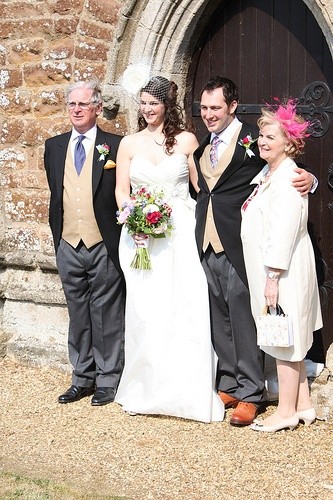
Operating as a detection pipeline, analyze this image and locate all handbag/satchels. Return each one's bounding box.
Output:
[256,302,293,347]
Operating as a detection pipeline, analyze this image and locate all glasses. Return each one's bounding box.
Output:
[67,100,89,109]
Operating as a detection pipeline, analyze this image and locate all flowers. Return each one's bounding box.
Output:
[116,184,172,270]
[96,143,110,162]
[102,158,116,170]
[238,133,260,161]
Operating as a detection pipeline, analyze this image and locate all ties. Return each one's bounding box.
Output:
[209,137,221,167]
[73,135,86,175]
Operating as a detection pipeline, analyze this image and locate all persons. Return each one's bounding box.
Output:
[114,76,226,423]
[239,97,323,431]
[194,77,332,426]
[44,82,132,405]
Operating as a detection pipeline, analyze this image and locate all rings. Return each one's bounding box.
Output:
[271,300,273,301]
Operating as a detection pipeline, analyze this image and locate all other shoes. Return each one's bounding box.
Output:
[263,378,280,401]
[303,359,323,377]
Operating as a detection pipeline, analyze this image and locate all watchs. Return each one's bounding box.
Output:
[267,272,280,279]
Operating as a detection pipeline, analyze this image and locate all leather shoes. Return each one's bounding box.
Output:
[90,387,114,405]
[217,391,237,408]
[59,386,93,403]
[229,401,257,425]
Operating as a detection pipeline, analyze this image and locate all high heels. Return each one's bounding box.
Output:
[296,407,316,427]
[250,413,298,431]
